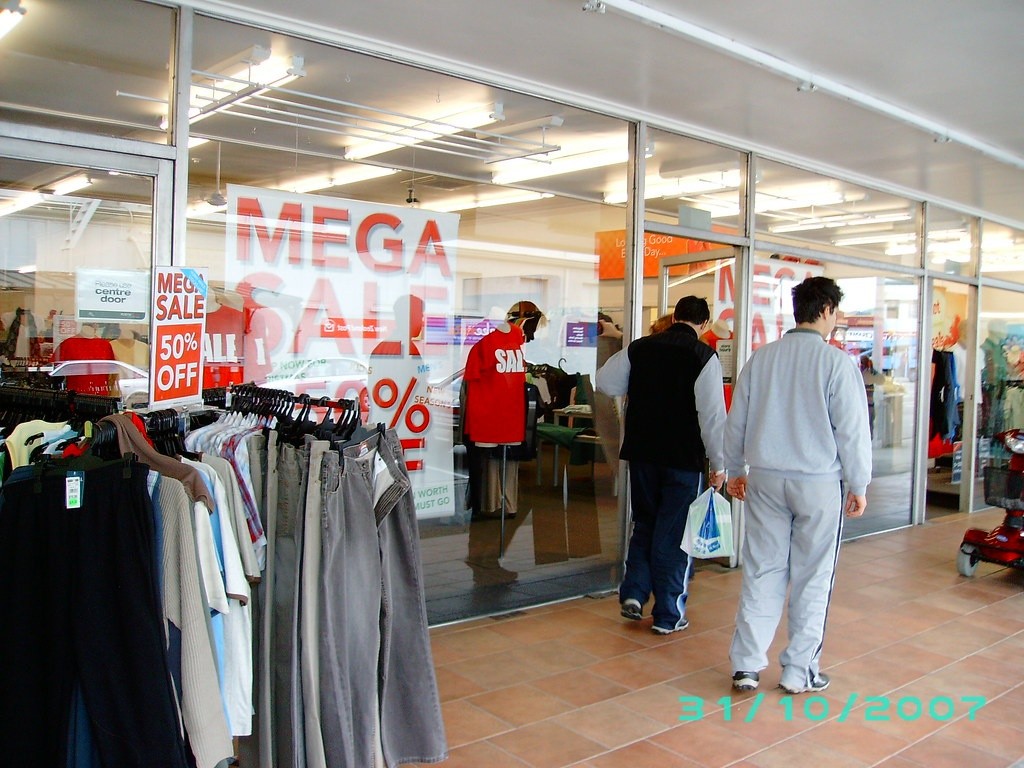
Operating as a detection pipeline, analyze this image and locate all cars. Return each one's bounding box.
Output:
[46,360,150,410]
[415,355,562,459]
[263,356,374,426]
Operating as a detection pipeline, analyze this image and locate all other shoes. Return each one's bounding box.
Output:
[473,567,518,584]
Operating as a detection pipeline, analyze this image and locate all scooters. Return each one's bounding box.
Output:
[954,428,1024,579]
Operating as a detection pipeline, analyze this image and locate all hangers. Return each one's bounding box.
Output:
[558,357,567,370]
[0,357,201,495]
[202,381,386,467]
[523,362,549,378]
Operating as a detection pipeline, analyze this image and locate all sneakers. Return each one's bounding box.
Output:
[620,598,644,620]
[802,672,830,693]
[731,671,760,690]
[650,618,690,634]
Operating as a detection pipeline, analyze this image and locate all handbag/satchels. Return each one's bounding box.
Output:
[679,486,735,559]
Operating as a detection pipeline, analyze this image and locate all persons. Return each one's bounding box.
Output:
[364,294,430,455]
[463,306,529,449]
[0,288,249,402]
[723,276,873,694]
[461,302,551,587]
[596,296,726,634]
